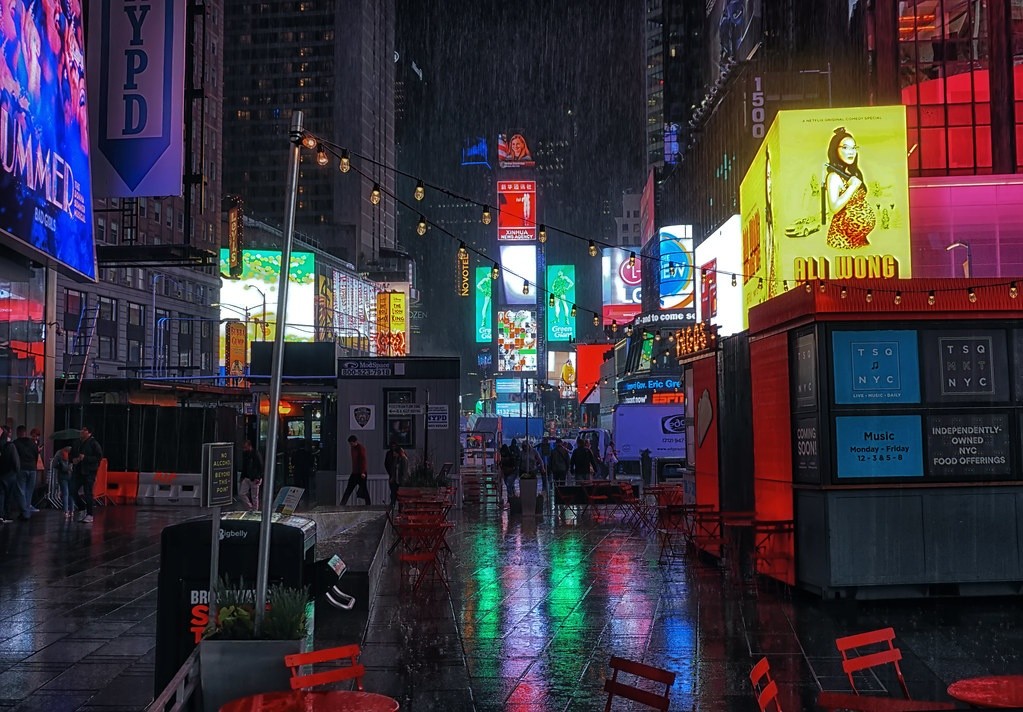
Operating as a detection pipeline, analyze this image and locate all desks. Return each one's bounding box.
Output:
[216,691,400,712]
[948,676,1023,709]
[720,518,759,582]
[656,502,716,566]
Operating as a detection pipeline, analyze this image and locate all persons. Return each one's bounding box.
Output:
[550,439,569,486]
[68,422,103,523]
[519,441,545,477]
[292,439,320,493]
[550,270,574,325]
[478,273,491,326]
[0,426,25,523]
[571,432,604,483]
[541,439,552,475]
[604,441,619,480]
[385,442,410,509]
[239,439,263,510]
[339,435,371,505]
[506,135,531,160]
[53,439,75,518]
[13,425,45,518]
[499,438,520,503]
[826,133,876,247]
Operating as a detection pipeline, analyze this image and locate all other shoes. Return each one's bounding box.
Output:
[82,515,93,523]
[77,510,87,521]
[0,513,14,523]
[29,505,40,512]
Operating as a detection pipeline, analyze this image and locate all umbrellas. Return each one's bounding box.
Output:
[50,429,83,439]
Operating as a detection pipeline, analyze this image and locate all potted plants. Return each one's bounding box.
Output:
[198,579,305,712]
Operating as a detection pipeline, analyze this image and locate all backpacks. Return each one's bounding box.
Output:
[0,442,15,476]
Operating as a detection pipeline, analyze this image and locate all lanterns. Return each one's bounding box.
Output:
[260,397,292,418]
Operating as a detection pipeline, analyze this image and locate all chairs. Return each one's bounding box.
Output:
[285,645,365,694]
[390,486,456,598]
[602,655,676,712]
[836,627,911,701]
[749,657,782,712]
[555,479,796,594]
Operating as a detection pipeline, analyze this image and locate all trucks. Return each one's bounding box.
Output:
[571,403,686,482]
[463,416,544,466]
